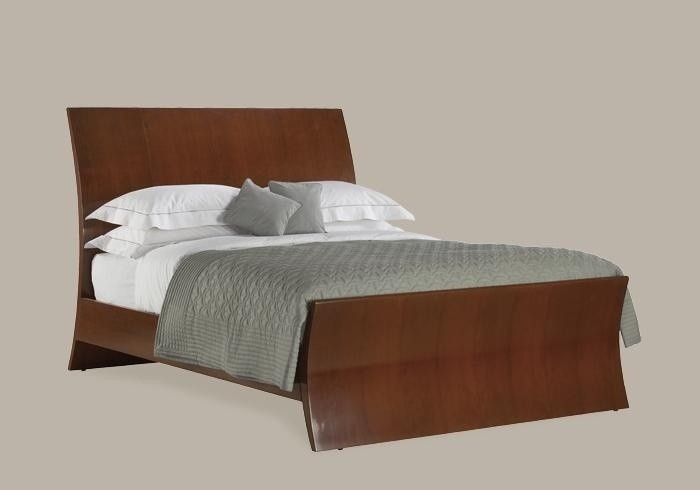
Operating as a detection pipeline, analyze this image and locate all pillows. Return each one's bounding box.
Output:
[82,225,240,262]
[265,178,416,220]
[222,177,305,239]
[84,182,242,231]
[269,179,329,237]
[320,219,405,236]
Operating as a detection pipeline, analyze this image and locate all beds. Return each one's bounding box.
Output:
[64,107,631,453]
